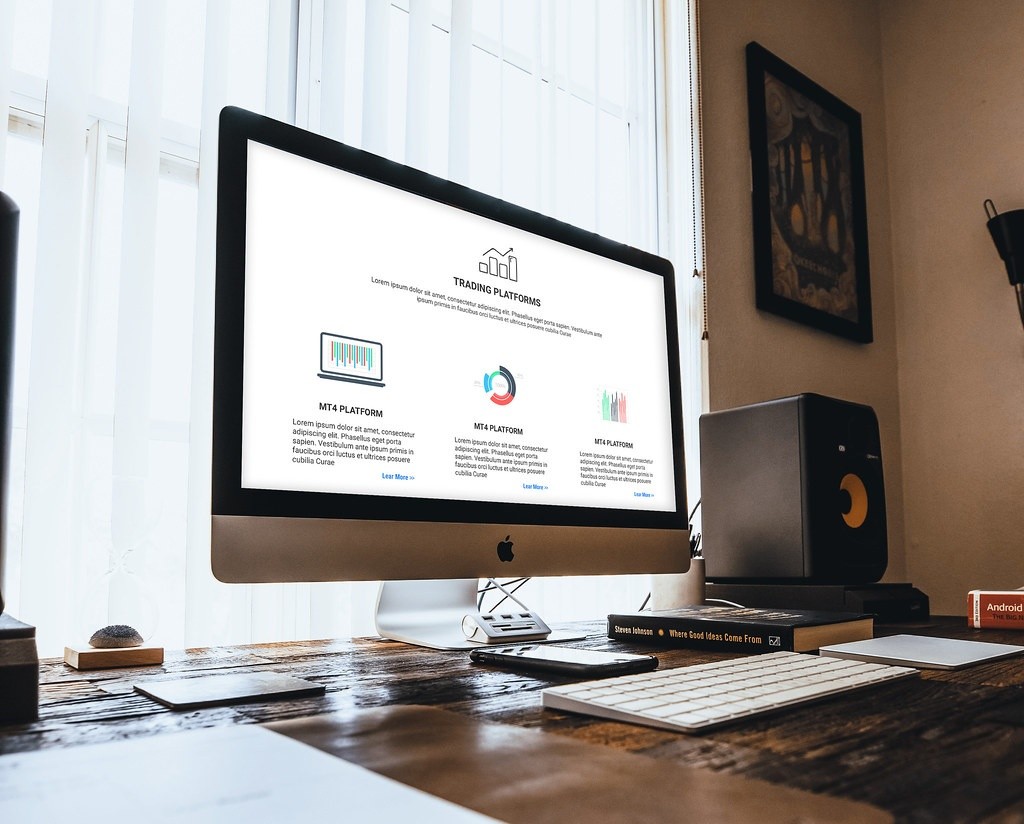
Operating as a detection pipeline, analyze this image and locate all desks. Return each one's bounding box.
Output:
[1,615,1024,824]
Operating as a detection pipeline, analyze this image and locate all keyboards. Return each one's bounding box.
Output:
[540,651,922,735]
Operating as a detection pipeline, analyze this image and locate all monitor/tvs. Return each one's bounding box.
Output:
[208,106,692,650]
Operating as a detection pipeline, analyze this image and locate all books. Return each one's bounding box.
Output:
[606,605,877,655]
[820,633,1024,671]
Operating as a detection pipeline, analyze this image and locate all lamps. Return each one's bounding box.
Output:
[982,198,1024,328]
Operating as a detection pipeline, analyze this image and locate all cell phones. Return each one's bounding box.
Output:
[470,644,659,680]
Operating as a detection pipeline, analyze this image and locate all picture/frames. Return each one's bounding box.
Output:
[745,40,874,346]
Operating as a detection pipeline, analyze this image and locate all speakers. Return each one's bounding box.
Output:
[699,392,890,585]
